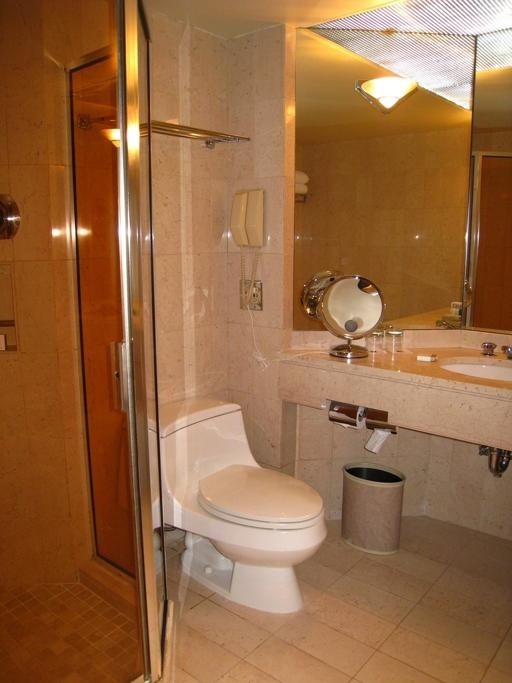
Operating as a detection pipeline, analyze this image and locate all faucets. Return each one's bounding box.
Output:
[501,346,512,359]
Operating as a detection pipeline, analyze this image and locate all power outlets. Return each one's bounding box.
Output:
[240,280,262,310]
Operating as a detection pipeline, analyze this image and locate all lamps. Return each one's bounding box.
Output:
[355,62,421,111]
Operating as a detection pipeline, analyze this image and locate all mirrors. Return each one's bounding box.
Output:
[292,27,512,335]
[319,275,384,358]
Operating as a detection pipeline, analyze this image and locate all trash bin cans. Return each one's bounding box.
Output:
[342,462,406,556]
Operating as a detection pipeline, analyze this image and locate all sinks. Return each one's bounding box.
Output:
[436,356,512,380]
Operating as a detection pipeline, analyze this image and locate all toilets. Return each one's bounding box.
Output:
[157,396,329,614]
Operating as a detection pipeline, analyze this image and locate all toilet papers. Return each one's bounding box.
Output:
[333,406,366,430]
